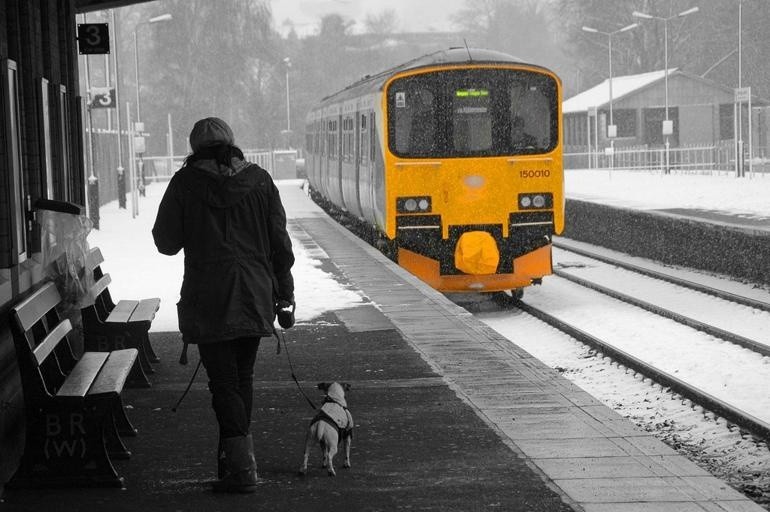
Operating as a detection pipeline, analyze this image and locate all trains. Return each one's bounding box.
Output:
[302,38,567,300]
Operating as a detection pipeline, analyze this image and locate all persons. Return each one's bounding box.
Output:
[151,115,298,494]
[513,115,538,150]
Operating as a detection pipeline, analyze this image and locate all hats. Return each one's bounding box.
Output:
[190,117,234,154]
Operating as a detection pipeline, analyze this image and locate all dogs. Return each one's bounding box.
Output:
[297,383,357,478]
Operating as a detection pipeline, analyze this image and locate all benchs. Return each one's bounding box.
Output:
[9,247,161,491]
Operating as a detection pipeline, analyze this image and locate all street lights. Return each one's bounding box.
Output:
[582,21,640,170]
[126,13,175,234]
[630,5,705,176]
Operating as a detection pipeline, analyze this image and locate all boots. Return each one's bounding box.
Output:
[212,431,260,493]
